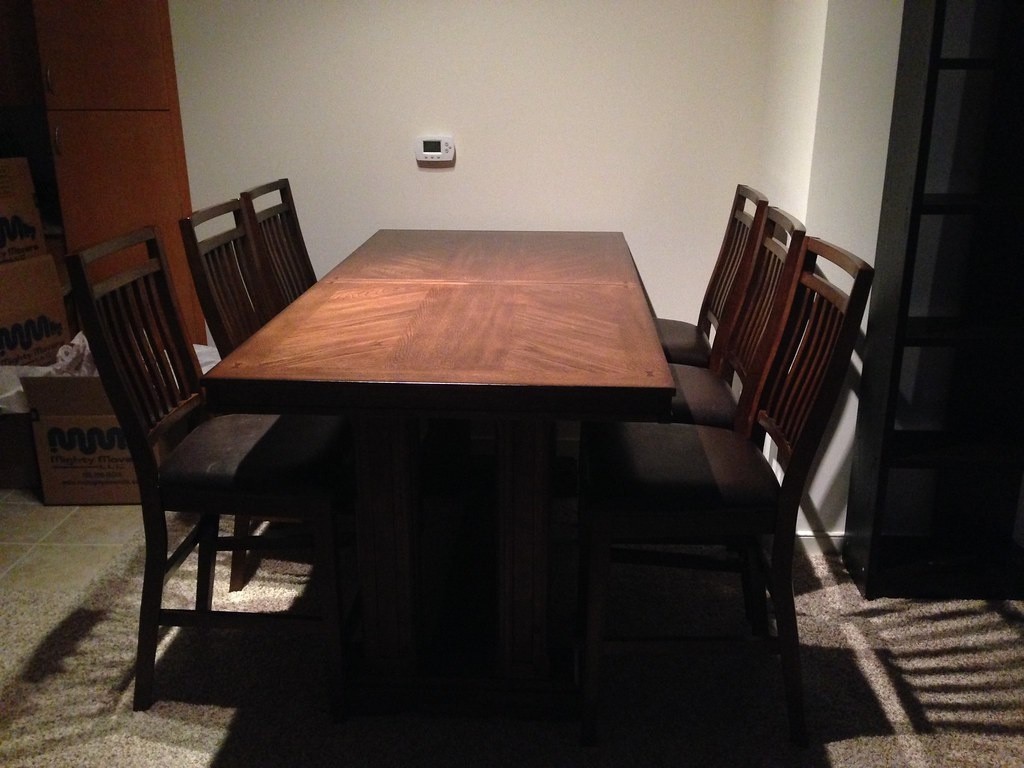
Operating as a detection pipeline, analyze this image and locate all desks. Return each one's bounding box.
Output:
[199,228,676,716]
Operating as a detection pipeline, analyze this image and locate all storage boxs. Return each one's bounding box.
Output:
[0,159,139,507]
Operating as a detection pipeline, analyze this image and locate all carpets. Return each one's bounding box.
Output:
[0,515,1024,766]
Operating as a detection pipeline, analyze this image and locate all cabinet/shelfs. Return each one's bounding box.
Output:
[33,0,206,344]
[861,0,1024,600]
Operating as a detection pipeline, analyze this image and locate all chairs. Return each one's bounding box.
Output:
[177,198,280,364]
[567,235,879,696]
[69,224,363,721]
[584,207,810,426]
[654,183,770,369]
[239,179,319,303]
